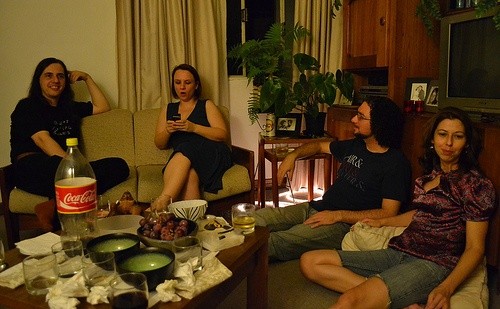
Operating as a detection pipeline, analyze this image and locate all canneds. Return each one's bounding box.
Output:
[265,113,275,137]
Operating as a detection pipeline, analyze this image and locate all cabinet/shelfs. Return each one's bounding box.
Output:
[326,0,500,269]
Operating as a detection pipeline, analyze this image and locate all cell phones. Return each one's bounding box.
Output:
[172,114,181,121]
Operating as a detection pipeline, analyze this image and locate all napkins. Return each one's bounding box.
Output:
[0,232,113,309]
[196,229,245,251]
[147,255,198,307]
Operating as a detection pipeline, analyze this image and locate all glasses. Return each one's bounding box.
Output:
[356,112,370,120]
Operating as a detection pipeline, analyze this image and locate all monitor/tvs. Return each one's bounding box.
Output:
[440,4,500,114]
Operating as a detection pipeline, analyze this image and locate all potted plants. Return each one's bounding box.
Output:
[227,20,354,138]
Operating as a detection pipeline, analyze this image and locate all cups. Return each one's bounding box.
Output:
[415,101,424,113]
[51,241,84,284]
[23,252,58,296]
[151,194,172,223]
[231,203,256,235]
[403,100,414,112]
[109,272,149,309]
[81,251,117,291]
[172,237,202,273]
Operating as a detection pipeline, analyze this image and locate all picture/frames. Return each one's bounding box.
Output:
[276,113,303,138]
[404,77,429,103]
[423,80,440,112]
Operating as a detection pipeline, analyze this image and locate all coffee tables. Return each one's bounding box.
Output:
[0,227,272,309]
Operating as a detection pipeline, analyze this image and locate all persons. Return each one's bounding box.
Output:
[254,97,413,267]
[414,86,438,105]
[300,108,494,309]
[147,64,225,217]
[279,120,294,131]
[10,58,130,236]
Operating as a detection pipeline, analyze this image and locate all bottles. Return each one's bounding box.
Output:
[265,112,276,137]
[55,138,99,240]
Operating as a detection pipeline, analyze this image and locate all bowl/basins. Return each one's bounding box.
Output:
[99,214,145,234]
[168,200,208,222]
[137,218,199,249]
[87,233,141,270]
[116,247,175,293]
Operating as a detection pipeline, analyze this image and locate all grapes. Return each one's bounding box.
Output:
[139,213,188,241]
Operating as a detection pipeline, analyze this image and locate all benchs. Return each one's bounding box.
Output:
[0,105,255,249]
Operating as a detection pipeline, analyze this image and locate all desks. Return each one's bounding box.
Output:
[255,132,336,209]
